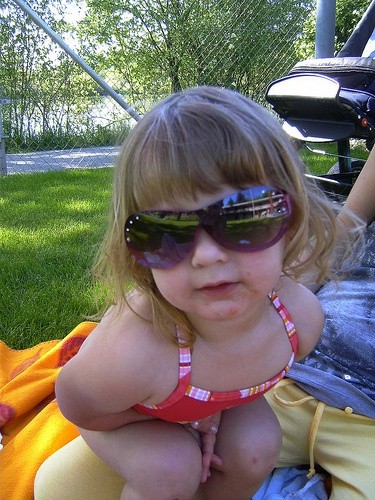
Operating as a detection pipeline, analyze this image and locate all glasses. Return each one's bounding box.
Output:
[123,187,297,271]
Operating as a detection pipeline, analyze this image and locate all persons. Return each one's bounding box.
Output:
[55,85,362,500]
[34,144,375,500]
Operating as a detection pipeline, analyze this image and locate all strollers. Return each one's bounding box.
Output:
[262,0,375,203]
